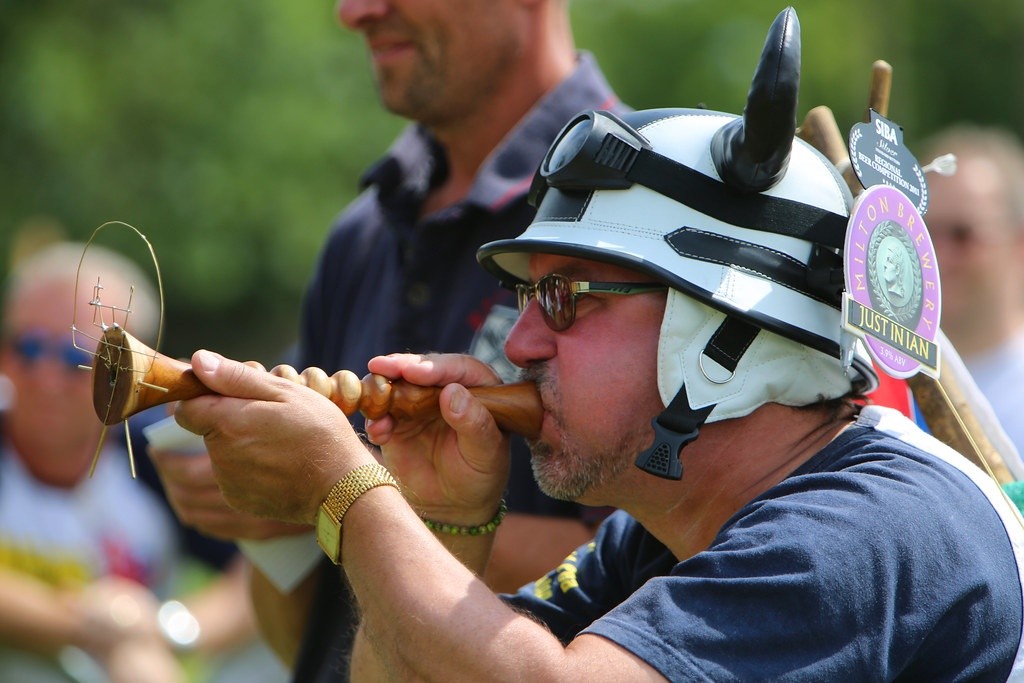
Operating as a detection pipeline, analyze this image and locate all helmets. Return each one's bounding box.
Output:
[477,6,880,479]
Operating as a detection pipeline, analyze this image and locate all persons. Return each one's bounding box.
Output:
[911,124,1024,470]
[146,0,637,683]
[0,241,290,683]
[174,4,1024,683]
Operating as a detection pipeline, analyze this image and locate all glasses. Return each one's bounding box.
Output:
[515,274,669,332]
[16,342,101,370]
[527,110,847,251]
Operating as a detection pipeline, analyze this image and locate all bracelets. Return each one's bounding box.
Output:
[422,498,507,535]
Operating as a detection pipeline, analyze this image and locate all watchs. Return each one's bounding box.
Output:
[317,464,401,565]
